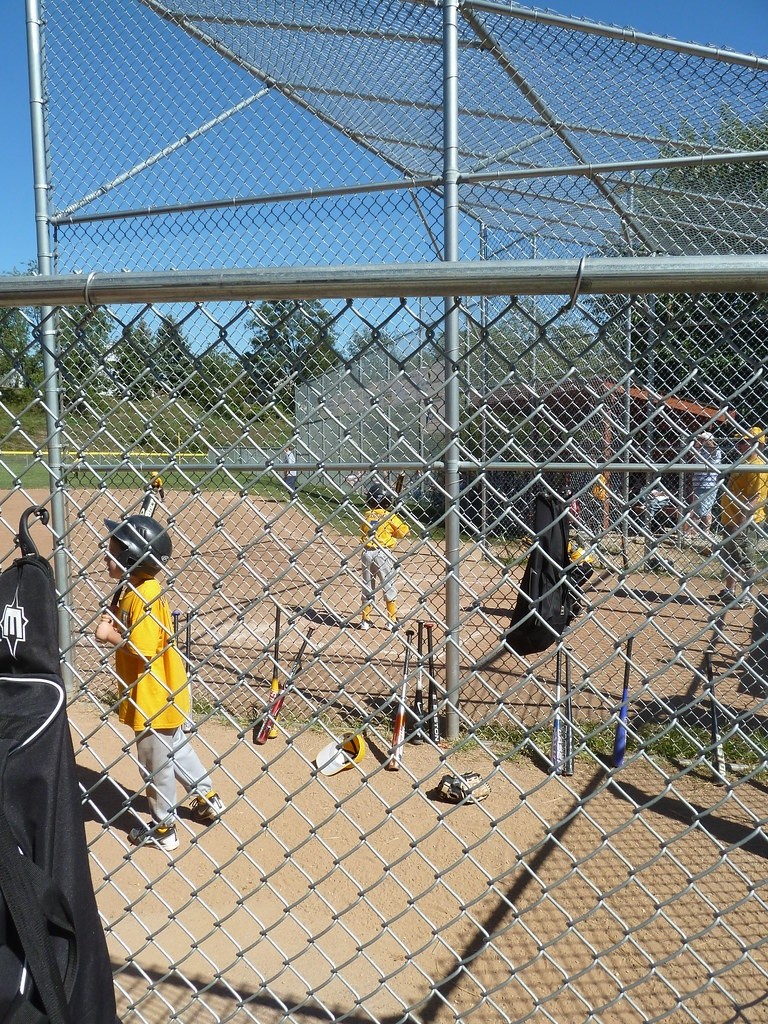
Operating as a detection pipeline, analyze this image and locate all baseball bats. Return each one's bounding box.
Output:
[95,488,160,645]
[423,623,440,745]
[171,610,193,733]
[551,639,574,777]
[703,650,727,787]
[388,629,414,771]
[411,619,425,745]
[254,603,315,745]
[393,471,406,515]
[611,633,635,767]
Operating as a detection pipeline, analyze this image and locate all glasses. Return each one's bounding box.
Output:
[738,437,747,443]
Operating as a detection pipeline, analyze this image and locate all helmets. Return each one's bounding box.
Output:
[366,483,392,509]
[104,515,172,577]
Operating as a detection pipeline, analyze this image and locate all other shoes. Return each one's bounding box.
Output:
[576,608,583,619]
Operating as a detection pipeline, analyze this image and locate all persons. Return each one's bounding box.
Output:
[149,472,165,500]
[683,435,721,539]
[359,483,411,631]
[284,447,298,502]
[96,515,225,850]
[706,427,768,607]
[567,542,594,617]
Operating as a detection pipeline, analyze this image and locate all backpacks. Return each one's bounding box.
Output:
[505,488,594,656]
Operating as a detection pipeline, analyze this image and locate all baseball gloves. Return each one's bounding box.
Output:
[520,537,533,551]
[435,770,491,804]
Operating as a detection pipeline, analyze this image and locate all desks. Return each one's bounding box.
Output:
[631,506,678,530]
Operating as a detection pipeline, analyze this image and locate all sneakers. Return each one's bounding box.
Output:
[709,589,735,600]
[188,792,225,822]
[725,594,753,609]
[129,820,180,852]
[385,621,405,630]
[360,618,374,630]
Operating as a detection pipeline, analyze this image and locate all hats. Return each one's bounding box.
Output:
[734,426,765,444]
[314,733,365,776]
[698,432,714,441]
